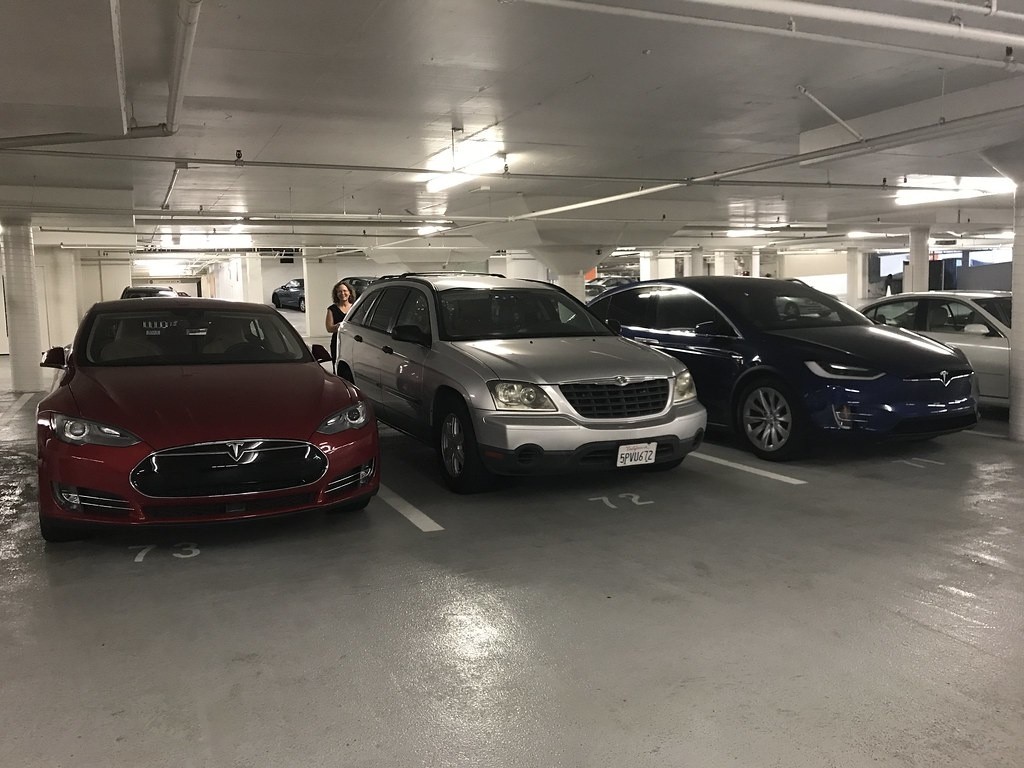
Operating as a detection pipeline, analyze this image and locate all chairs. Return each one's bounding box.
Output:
[100,317,254,360]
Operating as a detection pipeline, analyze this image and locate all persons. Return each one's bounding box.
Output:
[327,283,359,373]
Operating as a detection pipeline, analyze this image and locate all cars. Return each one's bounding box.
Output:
[34,296,380,541]
[339,274,380,298]
[585,277,634,295]
[585,275,982,461]
[334,271,708,495]
[271,278,306,312]
[856,290,1012,408]
[120,285,180,300]
[775,278,838,316]
